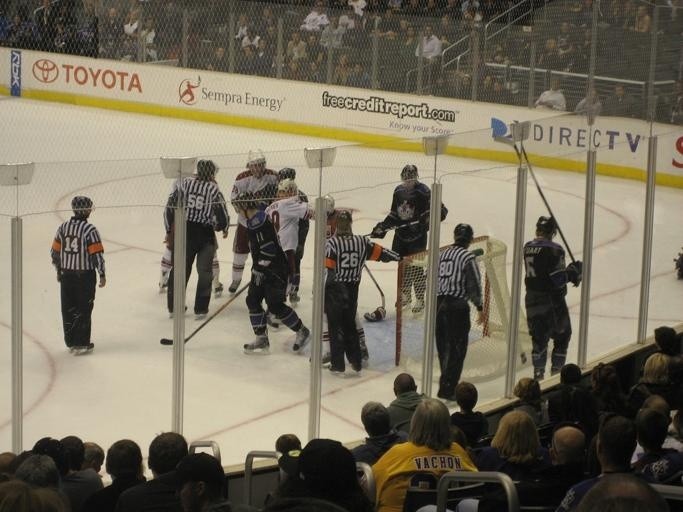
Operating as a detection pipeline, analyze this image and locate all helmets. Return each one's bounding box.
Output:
[536,217,558,233]
[72,197,93,218]
[277,167,298,196]
[246,149,266,164]
[337,209,353,230]
[197,159,215,178]
[454,224,473,245]
[325,195,335,212]
[401,164,418,180]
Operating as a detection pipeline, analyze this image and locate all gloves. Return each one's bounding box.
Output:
[566,260,582,287]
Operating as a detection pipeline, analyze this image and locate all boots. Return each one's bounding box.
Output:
[293,327,310,350]
[229,281,240,291]
[159,276,170,290]
[326,363,346,374]
[243,334,271,350]
[289,288,300,301]
[215,283,223,297]
[438,389,462,400]
[351,363,365,373]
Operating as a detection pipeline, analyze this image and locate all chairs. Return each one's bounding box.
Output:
[0,1,682,128]
[253,350,682,512]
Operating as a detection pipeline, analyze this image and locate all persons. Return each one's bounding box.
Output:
[524,216,582,382]
[1,0,682,126]
[50,196,106,354]
[1,327,683,511]
[162,156,485,404]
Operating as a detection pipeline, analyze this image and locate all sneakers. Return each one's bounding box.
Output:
[66,343,94,350]
[395,293,425,313]
[167,303,189,316]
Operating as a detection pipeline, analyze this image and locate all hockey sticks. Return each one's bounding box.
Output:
[160,280,252,345]
[495,135,575,264]
[364,264,385,308]
